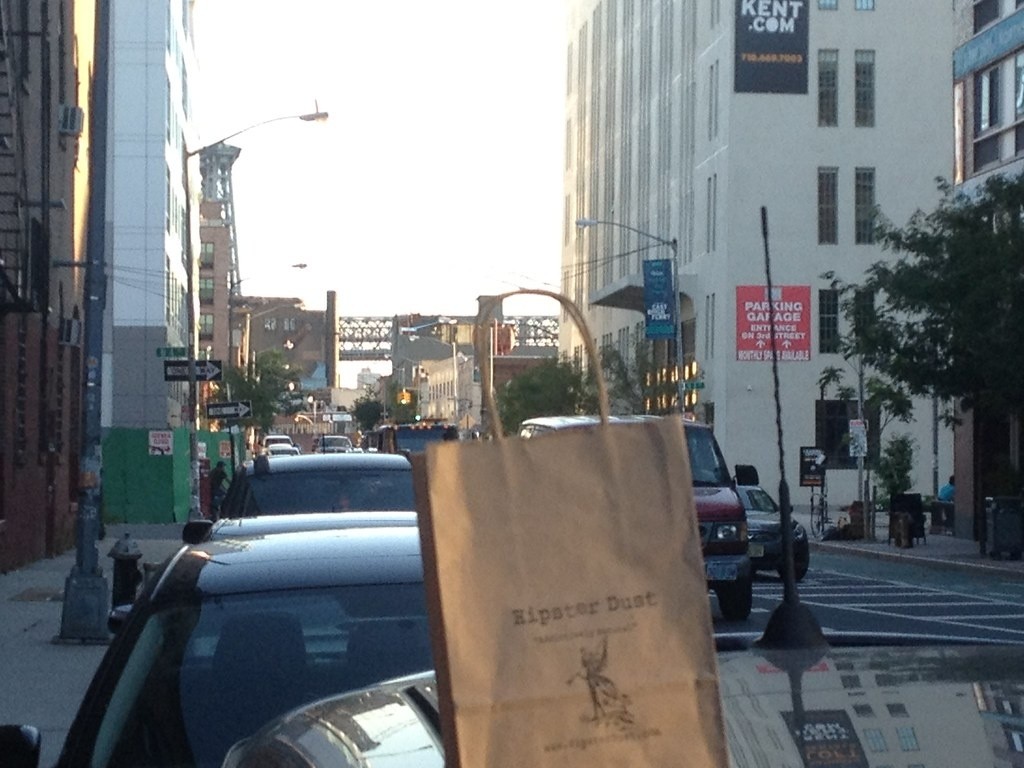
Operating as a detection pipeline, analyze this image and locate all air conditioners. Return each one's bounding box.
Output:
[59,104,83,136]
[59,317,80,345]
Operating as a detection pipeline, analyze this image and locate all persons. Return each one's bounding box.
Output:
[937,474,955,505]
[207,461,232,523]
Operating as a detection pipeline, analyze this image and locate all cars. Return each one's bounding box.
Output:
[0,511,444,768]
[222,647,1024,768]
[216,452,418,521]
[315,435,353,453]
[258,435,302,458]
[735,484,811,584]
[680,420,760,624]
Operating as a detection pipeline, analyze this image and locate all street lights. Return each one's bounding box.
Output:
[228,264,307,482]
[575,219,687,416]
[182,100,330,521]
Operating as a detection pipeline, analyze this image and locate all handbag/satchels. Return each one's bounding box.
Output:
[412,288,731,768]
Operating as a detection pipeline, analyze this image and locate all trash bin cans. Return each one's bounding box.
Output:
[984,494,1024,559]
